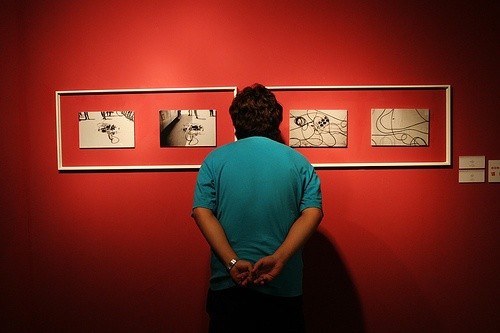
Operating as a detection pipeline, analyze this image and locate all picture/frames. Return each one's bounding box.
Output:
[55,87,237,170]
[264,85,451,167]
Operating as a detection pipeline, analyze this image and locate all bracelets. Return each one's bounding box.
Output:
[226,257,240,273]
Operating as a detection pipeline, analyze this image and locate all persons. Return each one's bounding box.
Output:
[191,83,324,333]
